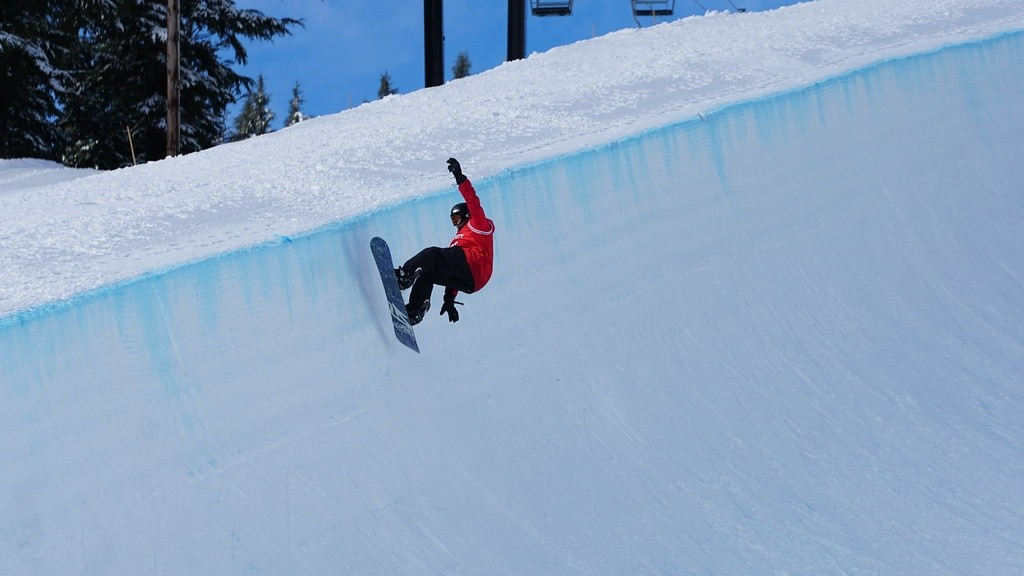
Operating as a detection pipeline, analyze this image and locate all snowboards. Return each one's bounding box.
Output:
[366,236,421,351]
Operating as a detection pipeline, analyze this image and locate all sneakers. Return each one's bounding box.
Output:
[394,266,414,290]
[409,299,431,326]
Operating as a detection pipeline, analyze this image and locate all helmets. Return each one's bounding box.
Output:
[449,203,470,226]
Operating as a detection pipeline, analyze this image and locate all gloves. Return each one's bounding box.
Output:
[446,158,467,185]
[440,294,464,323]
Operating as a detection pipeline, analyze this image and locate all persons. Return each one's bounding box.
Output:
[393,156,496,328]
